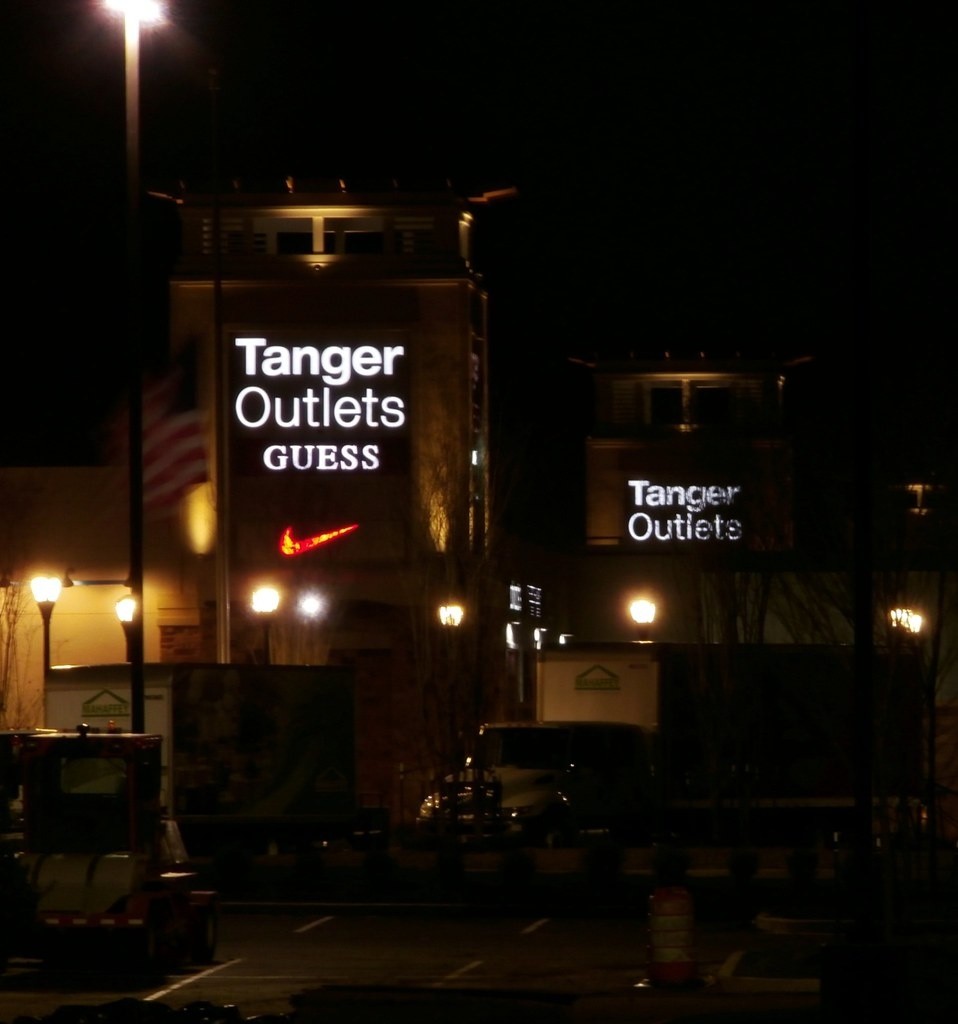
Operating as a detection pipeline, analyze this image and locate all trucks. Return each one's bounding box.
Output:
[416,640,926,848]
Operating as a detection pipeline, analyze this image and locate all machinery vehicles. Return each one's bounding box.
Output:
[0,737,220,990]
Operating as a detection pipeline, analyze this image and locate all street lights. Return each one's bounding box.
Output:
[104,2,154,734]
[32,576,62,670]
[116,599,132,663]
[252,588,278,663]
